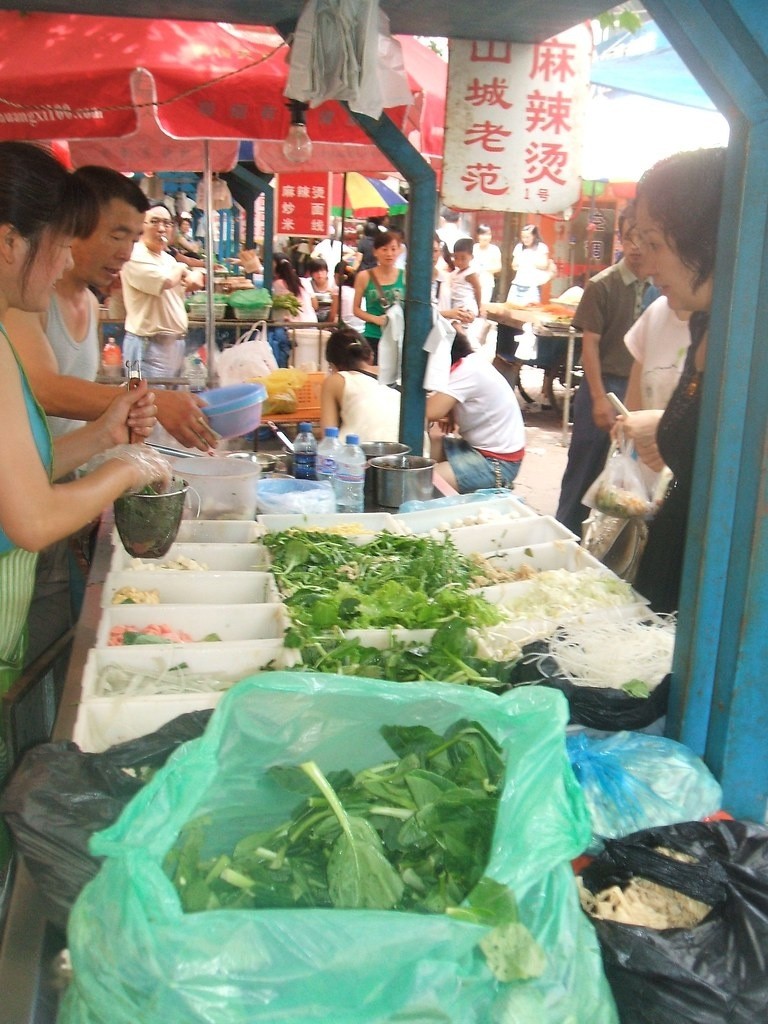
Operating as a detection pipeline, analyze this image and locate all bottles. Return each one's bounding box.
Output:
[316,427,344,489]
[181,358,207,390]
[333,434,366,513]
[103,337,121,378]
[293,423,318,480]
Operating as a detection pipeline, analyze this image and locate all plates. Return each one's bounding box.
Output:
[226,452,278,470]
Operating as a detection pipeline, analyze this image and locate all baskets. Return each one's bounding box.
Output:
[188,302,228,320]
[232,300,272,319]
[293,371,327,411]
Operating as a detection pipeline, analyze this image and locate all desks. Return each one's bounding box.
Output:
[487,304,583,447]
[0,450,720,1024]
[98,319,339,375]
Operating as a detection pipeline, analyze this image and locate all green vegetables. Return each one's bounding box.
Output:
[168,716,548,987]
[271,291,304,317]
[119,527,508,688]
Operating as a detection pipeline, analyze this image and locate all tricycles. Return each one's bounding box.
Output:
[486,298,588,425]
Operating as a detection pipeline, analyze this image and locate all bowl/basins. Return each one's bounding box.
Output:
[196,382,268,441]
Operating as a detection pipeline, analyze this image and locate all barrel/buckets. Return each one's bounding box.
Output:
[361,441,413,486]
[171,457,261,523]
[368,455,437,508]
[288,329,332,373]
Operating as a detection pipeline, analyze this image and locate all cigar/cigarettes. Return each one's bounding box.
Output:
[161,237,168,241]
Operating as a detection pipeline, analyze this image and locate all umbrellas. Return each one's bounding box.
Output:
[0,8,451,174]
[331,172,410,219]
[578,168,638,288]
[591,21,716,109]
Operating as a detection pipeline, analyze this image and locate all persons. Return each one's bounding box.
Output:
[0,139,206,989]
[225,148,729,621]
[321,328,401,444]
[450,238,482,337]
[472,225,501,303]
[506,225,549,304]
[426,333,525,491]
[556,207,666,537]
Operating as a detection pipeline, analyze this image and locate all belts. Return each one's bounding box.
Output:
[144,332,186,342]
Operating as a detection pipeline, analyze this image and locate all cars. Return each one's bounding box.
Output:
[289,239,325,278]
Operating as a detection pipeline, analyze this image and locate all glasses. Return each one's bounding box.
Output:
[145,219,175,226]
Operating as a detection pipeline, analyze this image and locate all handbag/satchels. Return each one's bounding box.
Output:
[581,436,666,516]
[1,709,216,930]
[568,729,723,854]
[246,368,306,416]
[215,320,277,387]
[61,668,616,1023]
[577,819,768,1024]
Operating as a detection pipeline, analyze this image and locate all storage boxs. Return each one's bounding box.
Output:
[110,544,272,575]
[68,695,222,797]
[79,640,303,710]
[95,602,293,650]
[258,496,676,687]
[102,569,278,608]
[111,519,261,548]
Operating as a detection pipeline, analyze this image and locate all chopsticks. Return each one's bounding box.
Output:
[606,392,630,417]
